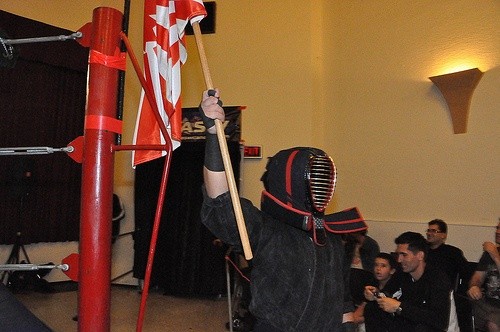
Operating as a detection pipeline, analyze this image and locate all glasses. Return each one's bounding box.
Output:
[426,227,444,234]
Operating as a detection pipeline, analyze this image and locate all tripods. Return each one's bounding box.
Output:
[0,197,31,287]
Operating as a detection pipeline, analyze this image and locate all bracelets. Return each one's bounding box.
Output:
[393,306,402,317]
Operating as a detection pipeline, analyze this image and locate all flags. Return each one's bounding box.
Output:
[132,0,207,169]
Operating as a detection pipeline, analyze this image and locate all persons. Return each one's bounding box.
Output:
[199,88,368,332]
[342,220,500,332]
[72,192,126,321]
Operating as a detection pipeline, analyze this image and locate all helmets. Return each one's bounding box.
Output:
[266,147,337,218]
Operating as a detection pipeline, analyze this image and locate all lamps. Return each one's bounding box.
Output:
[429,67,484,135]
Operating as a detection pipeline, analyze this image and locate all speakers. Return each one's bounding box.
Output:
[7,158,34,197]
